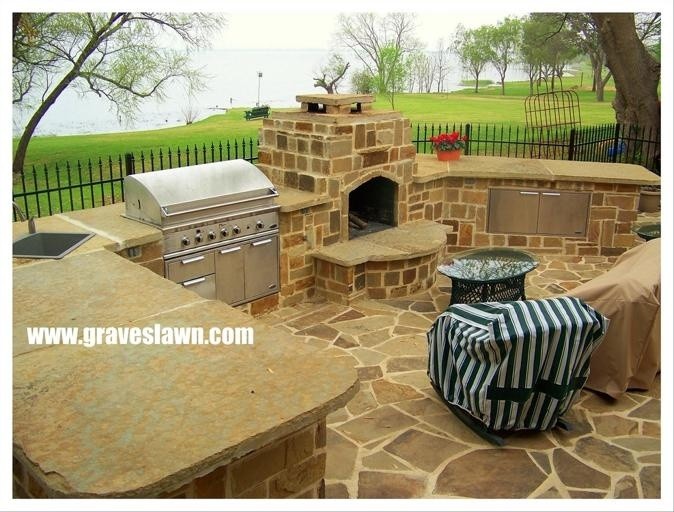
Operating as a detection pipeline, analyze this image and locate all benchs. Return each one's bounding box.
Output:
[245,106,270,121]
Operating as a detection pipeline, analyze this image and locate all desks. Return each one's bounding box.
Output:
[437,245,542,306]
[630,221,661,242]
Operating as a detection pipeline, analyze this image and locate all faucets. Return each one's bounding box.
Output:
[13,202,25,222]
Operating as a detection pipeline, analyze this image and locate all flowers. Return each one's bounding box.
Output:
[429,132,467,154]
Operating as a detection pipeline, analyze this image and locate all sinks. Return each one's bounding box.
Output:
[13,232,96,259]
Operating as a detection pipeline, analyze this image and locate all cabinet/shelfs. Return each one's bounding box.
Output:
[488,188,590,237]
[215,232,280,307]
[165,249,215,300]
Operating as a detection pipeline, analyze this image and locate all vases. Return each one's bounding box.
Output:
[436,148,461,161]
